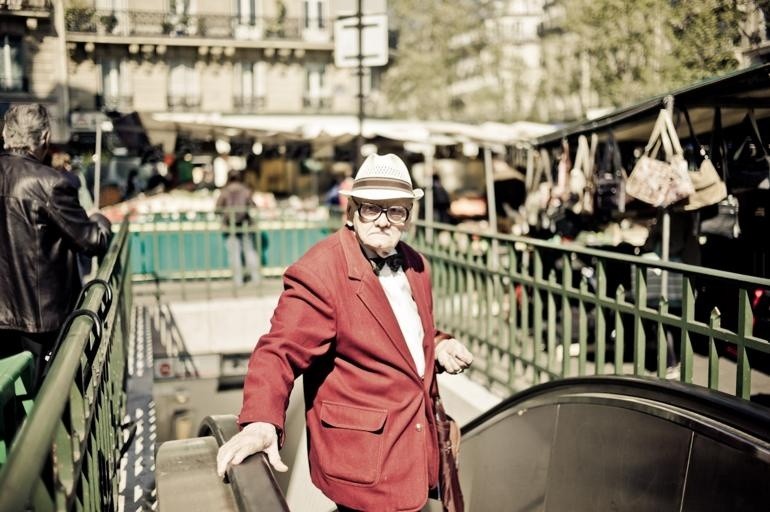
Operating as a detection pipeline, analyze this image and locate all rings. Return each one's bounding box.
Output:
[456,369,463,373]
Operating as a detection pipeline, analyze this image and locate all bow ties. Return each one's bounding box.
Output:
[369,254,403,273]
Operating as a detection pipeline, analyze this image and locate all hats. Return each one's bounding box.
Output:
[334,153,427,201]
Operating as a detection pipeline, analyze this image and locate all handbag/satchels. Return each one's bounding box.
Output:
[515,131,619,230]
[698,194,744,240]
[629,256,688,304]
[657,154,696,208]
[680,154,729,212]
[433,397,465,512]
[625,154,676,207]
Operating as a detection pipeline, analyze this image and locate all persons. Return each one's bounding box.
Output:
[46,139,454,229]
[214,149,479,512]
[214,167,263,291]
[0,96,113,439]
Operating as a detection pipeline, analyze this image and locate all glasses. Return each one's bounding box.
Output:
[350,195,415,225]
